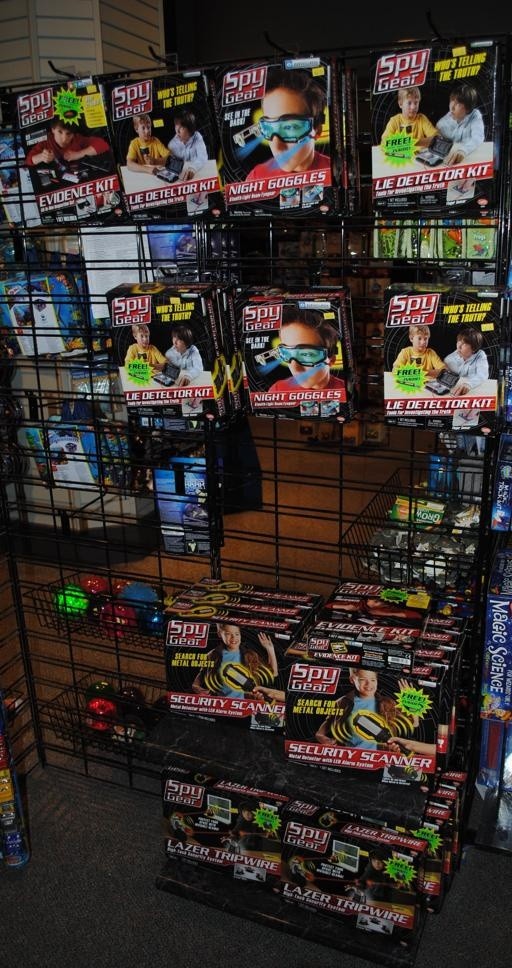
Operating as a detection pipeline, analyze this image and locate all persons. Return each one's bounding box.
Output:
[442,326,489,397]
[220,800,273,852]
[381,84,442,154]
[384,736,436,756]
[267,308,344,392]
[192,622,280,700]
[125,113,174,175]
[243,71,333,194]
[392,324,449,386]
[315,668,419,752]
[25,118,110,169]
[165,326,204,387]
[167,109,210,182]
[125,321,171,379]
[351,849,400,903]
[432,84,487,166]
[252,685,286,702]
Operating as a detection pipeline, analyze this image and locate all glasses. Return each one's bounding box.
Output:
[277,344,338,368]
[259,113,317,144]
